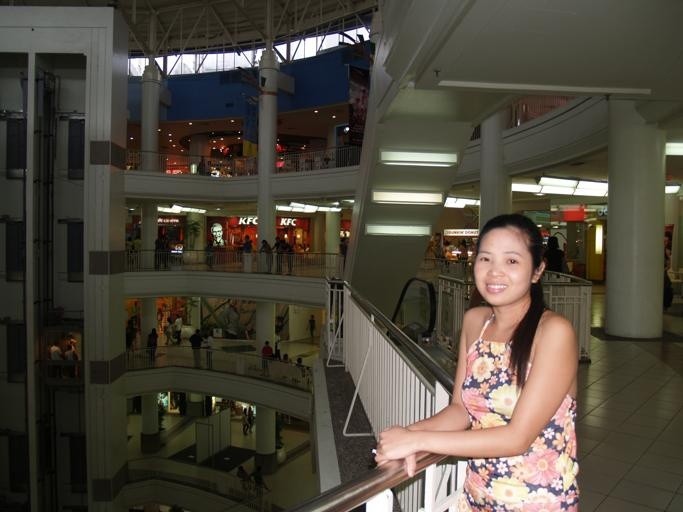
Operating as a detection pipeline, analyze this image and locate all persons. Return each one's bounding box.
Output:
[238,466,267,500]
[126,228,311,277]
[194,153,207,175]
[664,231,672,250]
[241,407,255,435]
[372,212,581,511]
[124,312,311,388]
[425,229,476,275]
[661,245,673,312]
[48,334,78,361]
[178,397,187,416]
[542,236,566,277]
[158,406,165,431]
